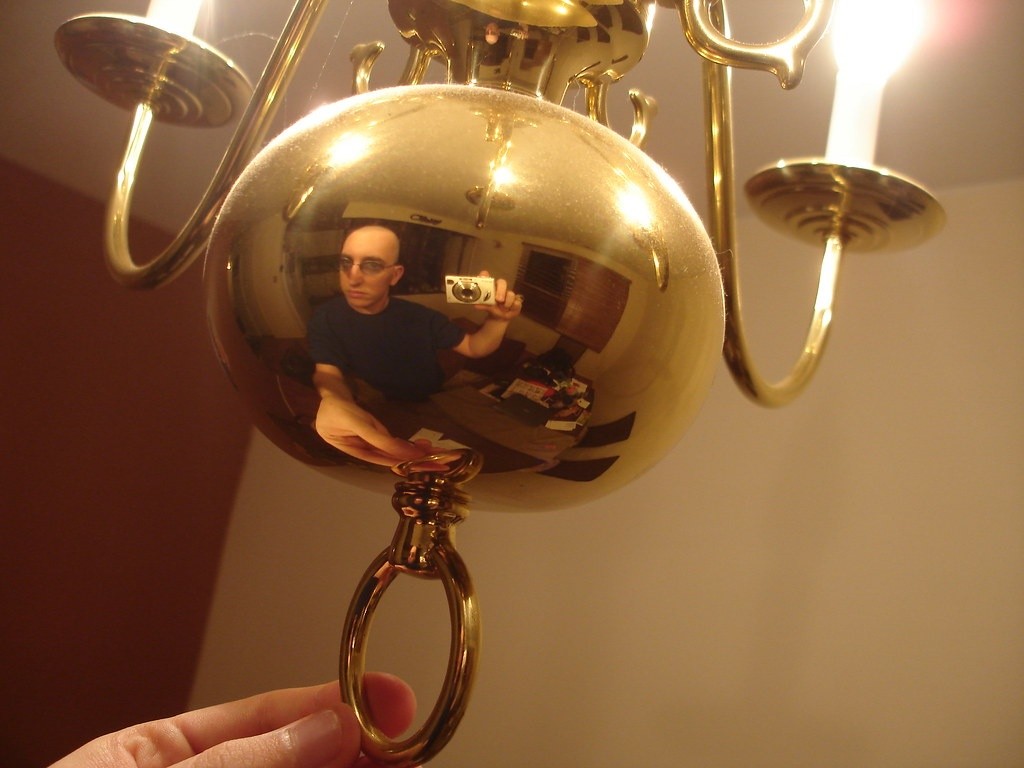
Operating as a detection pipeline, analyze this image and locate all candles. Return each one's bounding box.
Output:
[147,0,203,35]
[827,56,882,168]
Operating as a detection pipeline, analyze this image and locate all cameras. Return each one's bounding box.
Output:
[445,276,496,305]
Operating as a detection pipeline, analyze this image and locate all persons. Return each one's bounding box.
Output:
[45,672,424,768]
[310,225,523,471]
